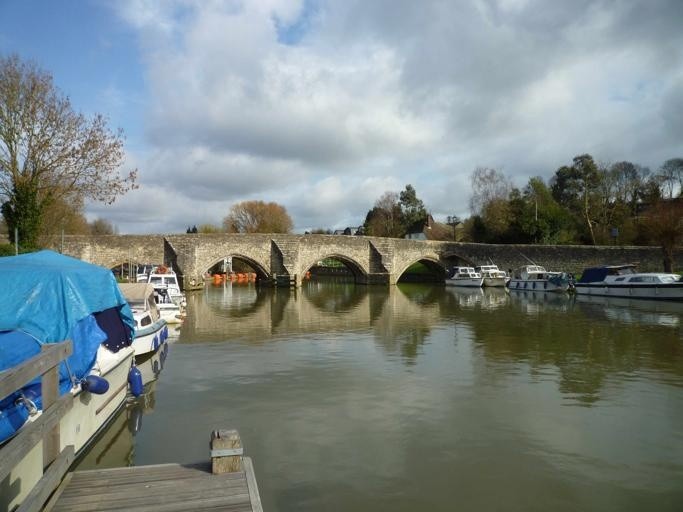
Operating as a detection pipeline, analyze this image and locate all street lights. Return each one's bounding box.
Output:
[446,216,458,240]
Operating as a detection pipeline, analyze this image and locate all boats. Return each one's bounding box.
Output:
[445,254,572,292]
[110,265,185,385]
[572,264,681,300]
[1,251,135,510]
